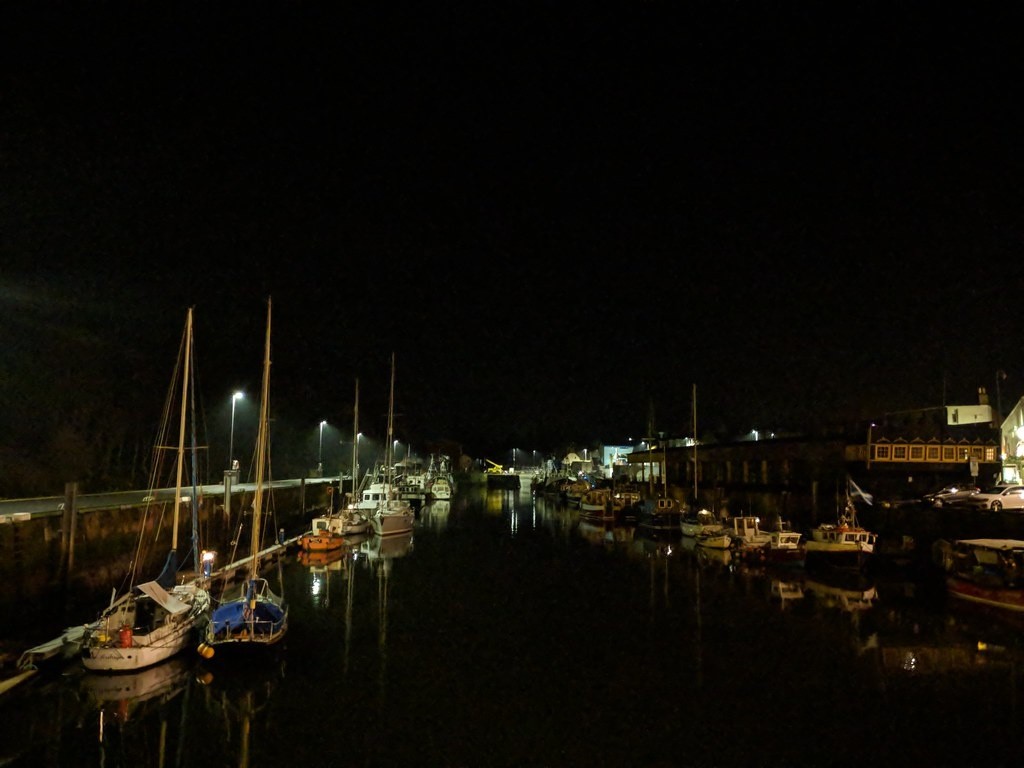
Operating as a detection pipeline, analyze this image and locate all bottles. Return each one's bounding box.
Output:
[119,623,133,648]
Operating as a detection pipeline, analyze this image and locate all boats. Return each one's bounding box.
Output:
[537,475,802,561]
[924,536,1024,626]
[302,374,457,551]
[801,521,877,565]
[76,307,225,673]
[203,295,295,644]
